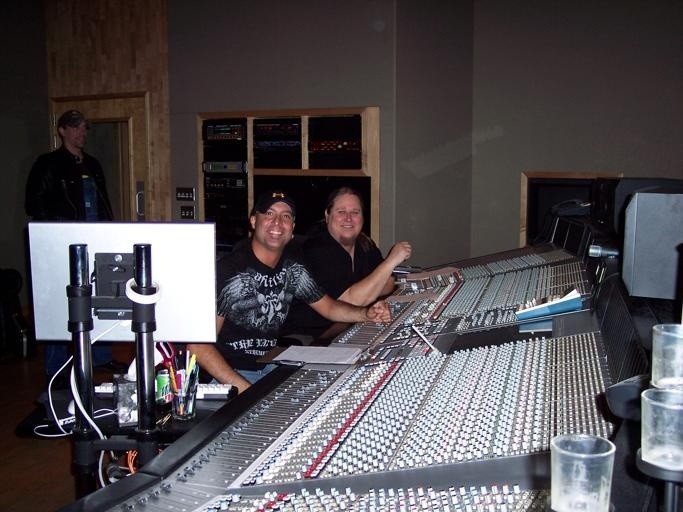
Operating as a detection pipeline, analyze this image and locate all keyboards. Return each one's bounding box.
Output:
[95,381,233,401]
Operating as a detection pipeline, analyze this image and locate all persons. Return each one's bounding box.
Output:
[183,184,394,401]
[21,108,127,391]
[285,184,412,349]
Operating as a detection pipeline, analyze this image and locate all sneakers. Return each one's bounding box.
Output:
[94,359,128,374]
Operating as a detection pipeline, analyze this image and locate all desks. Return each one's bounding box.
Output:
[16,360,228,499]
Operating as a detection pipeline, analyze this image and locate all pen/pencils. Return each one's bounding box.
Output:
[412,324,436,350]
[168,350,199,416]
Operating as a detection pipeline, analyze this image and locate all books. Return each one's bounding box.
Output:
[515,287,583,321]
[518,320,552,333]
[271,345,362,364]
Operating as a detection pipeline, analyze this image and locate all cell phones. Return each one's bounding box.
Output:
[279,360,305,367]
[395,265,422,272]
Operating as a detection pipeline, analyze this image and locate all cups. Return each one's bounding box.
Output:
[551,325,683,512]
[171,385,198,420]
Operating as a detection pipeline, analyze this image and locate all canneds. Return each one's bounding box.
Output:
[156,369,174,405]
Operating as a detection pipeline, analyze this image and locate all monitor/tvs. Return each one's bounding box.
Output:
[28,222,217,345]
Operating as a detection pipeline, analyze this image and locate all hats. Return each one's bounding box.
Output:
[248,189,295,217]
[56,110,88,129]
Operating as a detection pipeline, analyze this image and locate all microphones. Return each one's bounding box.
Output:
[588,244,620,258]
